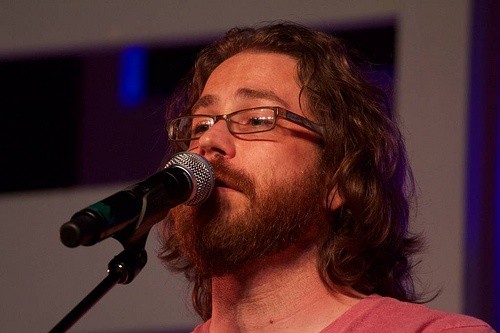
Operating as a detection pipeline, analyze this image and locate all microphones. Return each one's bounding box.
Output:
[59,152,215,249]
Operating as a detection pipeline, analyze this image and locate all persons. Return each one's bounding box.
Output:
[154,23,496,333]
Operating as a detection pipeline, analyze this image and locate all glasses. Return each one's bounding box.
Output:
[164,104,335,146]
